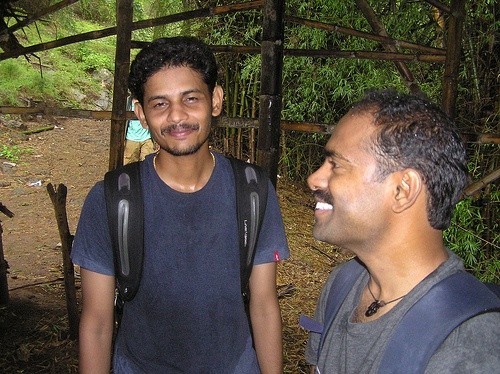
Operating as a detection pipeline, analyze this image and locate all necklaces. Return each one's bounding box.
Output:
[152,151,215,171]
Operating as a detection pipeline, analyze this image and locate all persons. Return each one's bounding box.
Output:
[300,94,499,374]
[70,34,294,374]
[365,279,408,317]
